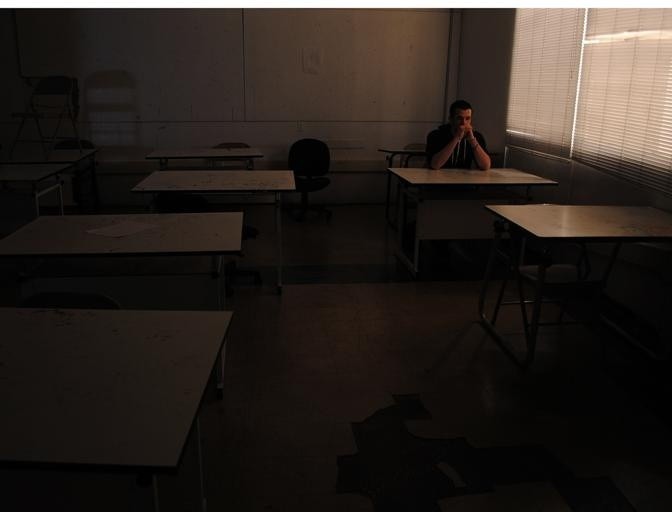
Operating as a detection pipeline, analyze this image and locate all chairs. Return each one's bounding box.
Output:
[287,138,333,223]
[10,75,85,157]
[209,142,255,170]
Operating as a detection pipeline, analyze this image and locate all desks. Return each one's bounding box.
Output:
[2,310,233,512]
[379,145,427,225]
[387,165,562,285]
[476,204,672,366]
[2,146,99,217]
[132,169,296,299]
[2,212,245,314]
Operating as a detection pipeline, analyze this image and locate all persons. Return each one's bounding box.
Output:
[422,98,492,273]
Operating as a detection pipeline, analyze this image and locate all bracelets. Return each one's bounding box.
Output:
[467,135,475,139]
[471,140,479,151]
[447,143,455,151]
[469,136,477,143]
